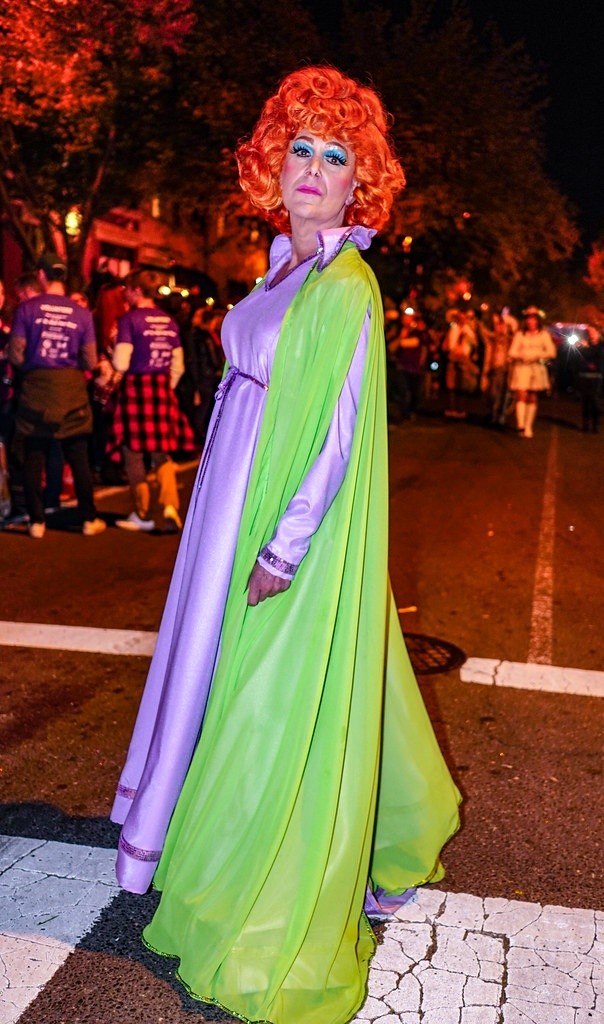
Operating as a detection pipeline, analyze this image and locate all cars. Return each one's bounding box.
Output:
[549,320,604,382]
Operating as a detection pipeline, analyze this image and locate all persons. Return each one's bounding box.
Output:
[109,66,463,1023]
[0,254,227,538]
[384,301,556,439]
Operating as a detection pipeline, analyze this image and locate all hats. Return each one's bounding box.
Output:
[521,306,547,319]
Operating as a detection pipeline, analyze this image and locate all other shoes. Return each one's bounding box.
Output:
[28,521,46,538]
[83,518,106,535]
[592,426,598,433]
[116,512,154,531]
[444,408,467,417]
[4,510,29,523]
[582,427,589,432]
[43,497,61,513]
[516,421,534,439]
[163,506,183,531]
